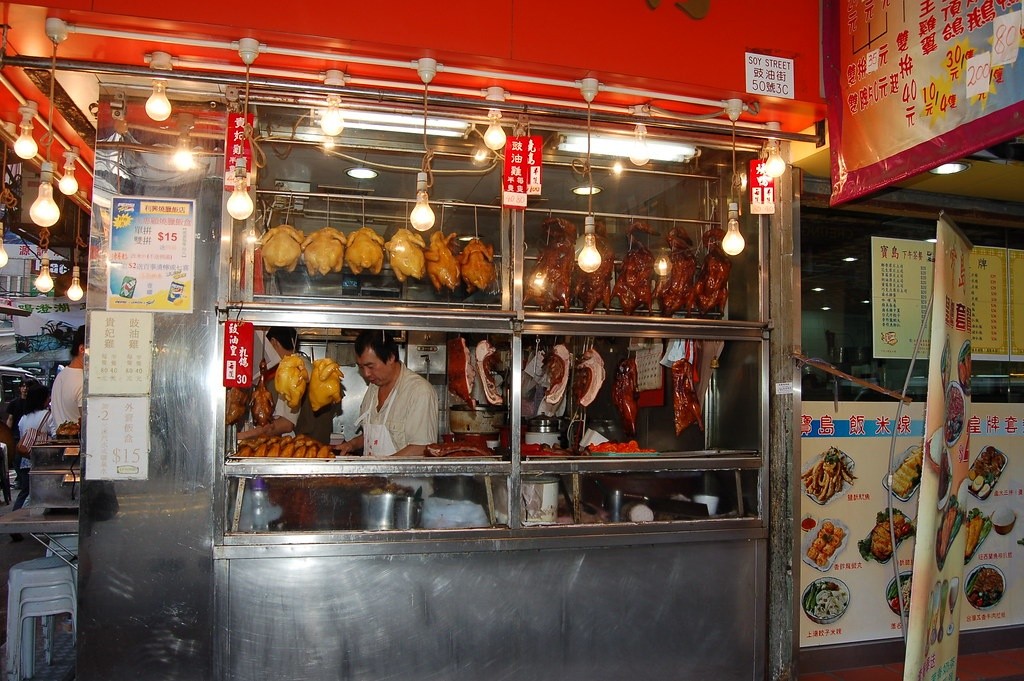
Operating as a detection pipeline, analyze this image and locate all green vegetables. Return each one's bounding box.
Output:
[967,507,991,543]
[967,567,1003,607]
[981,470,998,485]
[858,507,917,564]
[803,582,822,613]
[888,574,910,597]
[822,447,840,464]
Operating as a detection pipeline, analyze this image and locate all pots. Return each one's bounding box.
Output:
[492,478,560,526]
[449,401,623,454]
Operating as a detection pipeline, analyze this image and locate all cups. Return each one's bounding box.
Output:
[694,495,718,517]
[607,490,624,522]
[925,577,959,656]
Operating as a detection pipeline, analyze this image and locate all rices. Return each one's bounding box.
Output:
[990,506,1016,526]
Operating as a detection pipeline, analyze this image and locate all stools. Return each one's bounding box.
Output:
[6,535,79,681]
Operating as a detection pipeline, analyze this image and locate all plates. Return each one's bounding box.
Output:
[862,509,911,563]
[801,451,854,503]
[801,518,851,574]
[881,445,924,501]
[969,445,1007,501]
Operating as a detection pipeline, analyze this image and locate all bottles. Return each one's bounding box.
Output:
[250,479,269,531]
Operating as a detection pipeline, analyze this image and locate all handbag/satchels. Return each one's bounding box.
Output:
[16,411,53,459]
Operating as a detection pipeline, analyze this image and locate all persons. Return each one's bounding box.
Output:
[238,325,333,444]
[10,384,58,541]
[6,381,32,490]
[51,325,85,428]
[334,330,439,500]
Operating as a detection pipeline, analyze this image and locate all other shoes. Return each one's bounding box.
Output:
[10,480,20,489]
[9,533,23,542]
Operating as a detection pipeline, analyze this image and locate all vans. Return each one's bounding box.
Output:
[0,366,43,467]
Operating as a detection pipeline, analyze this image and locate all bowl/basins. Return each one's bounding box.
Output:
[944,381,966,447]
[937,446,953,509]
[964,564,1005,609]
[989,511,1017,535]
[800,577,850,623]
[885,570,912,616]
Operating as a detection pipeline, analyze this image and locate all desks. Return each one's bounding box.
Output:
[0,508,79,647]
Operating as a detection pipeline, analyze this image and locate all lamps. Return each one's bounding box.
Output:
[309,108,476,140]
[550,132,701,164]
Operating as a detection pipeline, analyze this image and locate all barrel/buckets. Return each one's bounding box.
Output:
[347,493,423,531]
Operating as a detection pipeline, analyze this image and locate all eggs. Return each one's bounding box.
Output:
[968,469,990,498]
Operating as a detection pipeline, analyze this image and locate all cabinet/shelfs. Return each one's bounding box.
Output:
[212,171,773,681]
